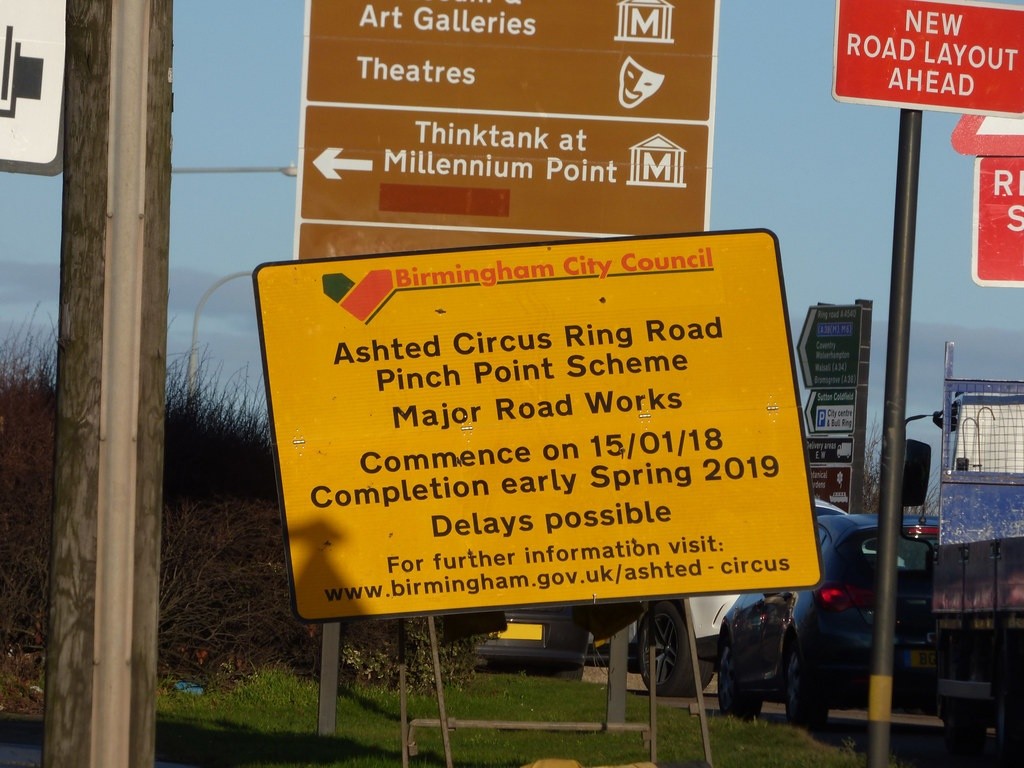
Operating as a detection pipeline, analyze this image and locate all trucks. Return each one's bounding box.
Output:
[885,338,1024,767]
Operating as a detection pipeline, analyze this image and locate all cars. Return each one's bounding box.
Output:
[456,600,591,679]
[714,510,999,737]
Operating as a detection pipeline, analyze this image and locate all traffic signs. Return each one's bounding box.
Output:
[800,463,851,511]
[795,304,861,390]
[803,388,858,436]
[295,0,723,267]
[803,438,854,467]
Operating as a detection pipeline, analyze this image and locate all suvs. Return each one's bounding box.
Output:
[634,497,851,693]
